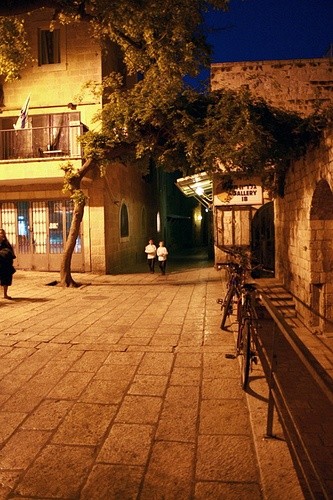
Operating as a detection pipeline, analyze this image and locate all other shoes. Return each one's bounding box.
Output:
[4,296,11,299]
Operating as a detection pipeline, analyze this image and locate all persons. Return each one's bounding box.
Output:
[0,228,16,299]
[157,241,168,276]
[145,239,157,274]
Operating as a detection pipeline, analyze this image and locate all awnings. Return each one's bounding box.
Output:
[177,169,215,211]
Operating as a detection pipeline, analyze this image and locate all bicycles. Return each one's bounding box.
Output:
[215,250,260,330]
[218,260,275,392]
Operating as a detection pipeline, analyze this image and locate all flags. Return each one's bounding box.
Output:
[14,96,31,129]
